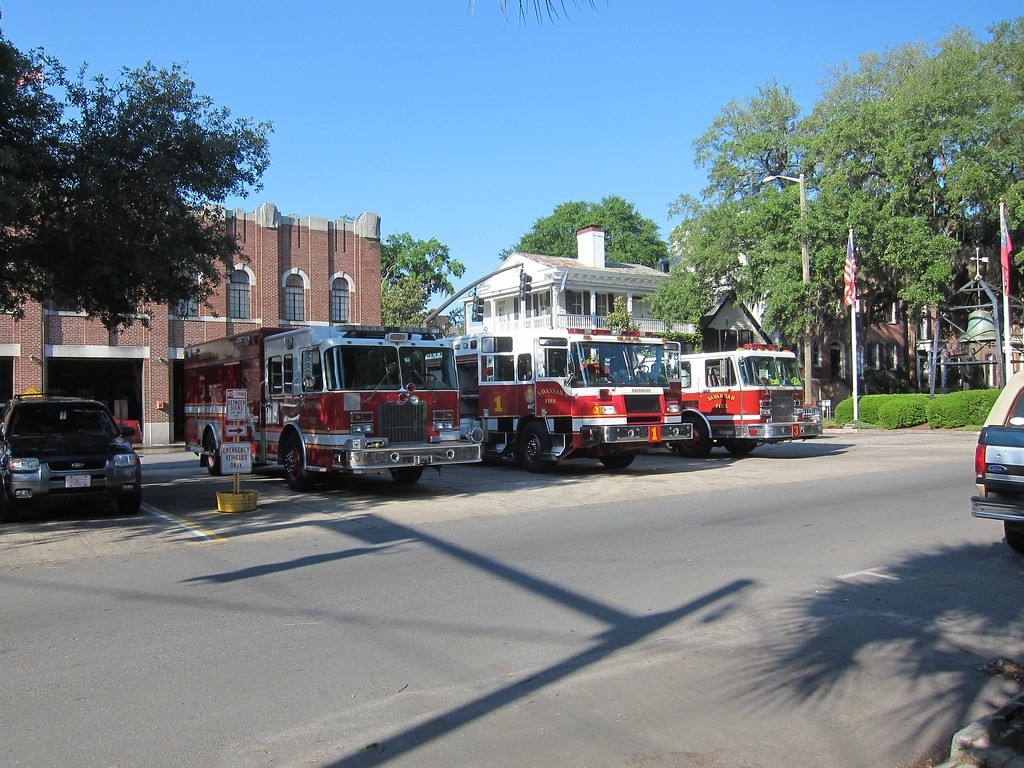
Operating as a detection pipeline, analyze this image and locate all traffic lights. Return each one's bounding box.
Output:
[471,296,484,322]
[518,273,533,301]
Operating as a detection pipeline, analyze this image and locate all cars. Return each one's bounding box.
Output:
[969,370,1024,552]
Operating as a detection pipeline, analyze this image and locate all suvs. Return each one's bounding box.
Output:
[0,392,144,518]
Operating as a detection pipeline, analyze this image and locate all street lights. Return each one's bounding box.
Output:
[761,173,813,406]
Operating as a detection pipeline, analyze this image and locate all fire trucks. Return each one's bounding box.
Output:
[183,323,485,495]
[404,326,694,473]
[581,342,824,458]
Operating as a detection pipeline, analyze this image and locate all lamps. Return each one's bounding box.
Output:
[29,355,34,361]
[157,357,163,364]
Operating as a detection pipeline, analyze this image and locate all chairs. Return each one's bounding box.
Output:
[608,358,627,381]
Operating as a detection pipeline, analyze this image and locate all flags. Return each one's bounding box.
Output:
[844,229,858,308]
[1001,202,1013,297]
